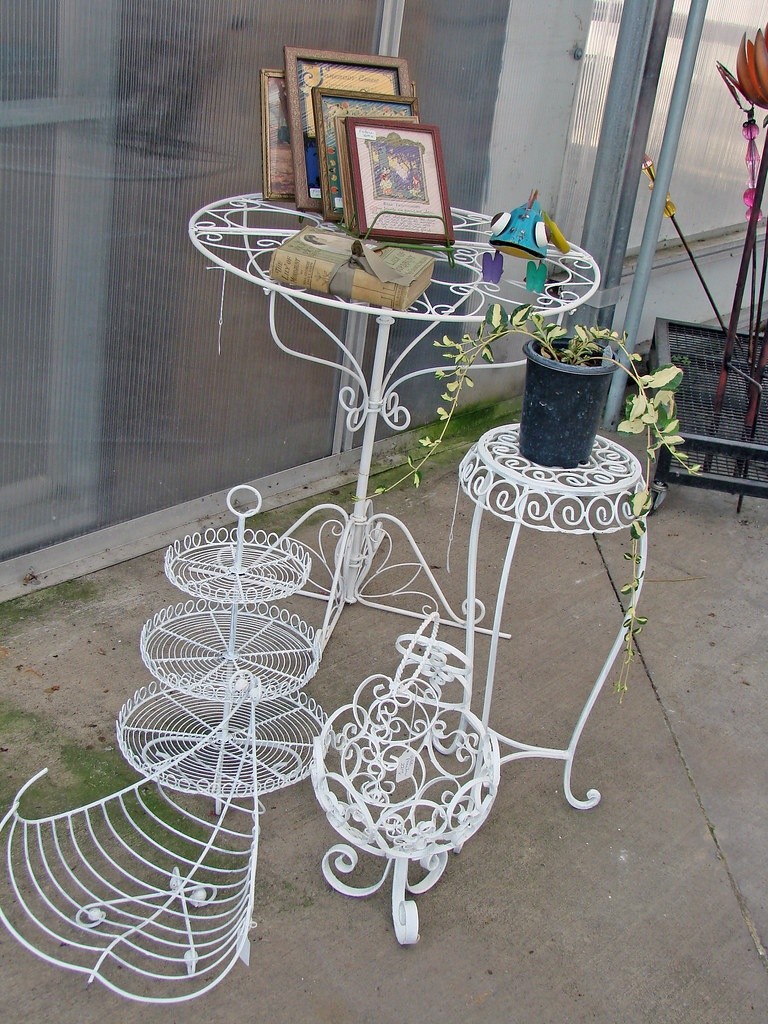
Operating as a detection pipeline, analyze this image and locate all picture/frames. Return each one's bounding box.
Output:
[311,84,419,223]
[333,113,420,231]
[261,66,417,206]
[280,46,412,209]
[344,116,456,245]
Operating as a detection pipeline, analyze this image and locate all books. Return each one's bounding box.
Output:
[270,225,434,312]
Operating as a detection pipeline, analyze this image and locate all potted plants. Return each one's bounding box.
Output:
[328,298,699,708]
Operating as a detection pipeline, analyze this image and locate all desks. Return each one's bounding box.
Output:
[187,193,599,689]
[443,427,650,809]
